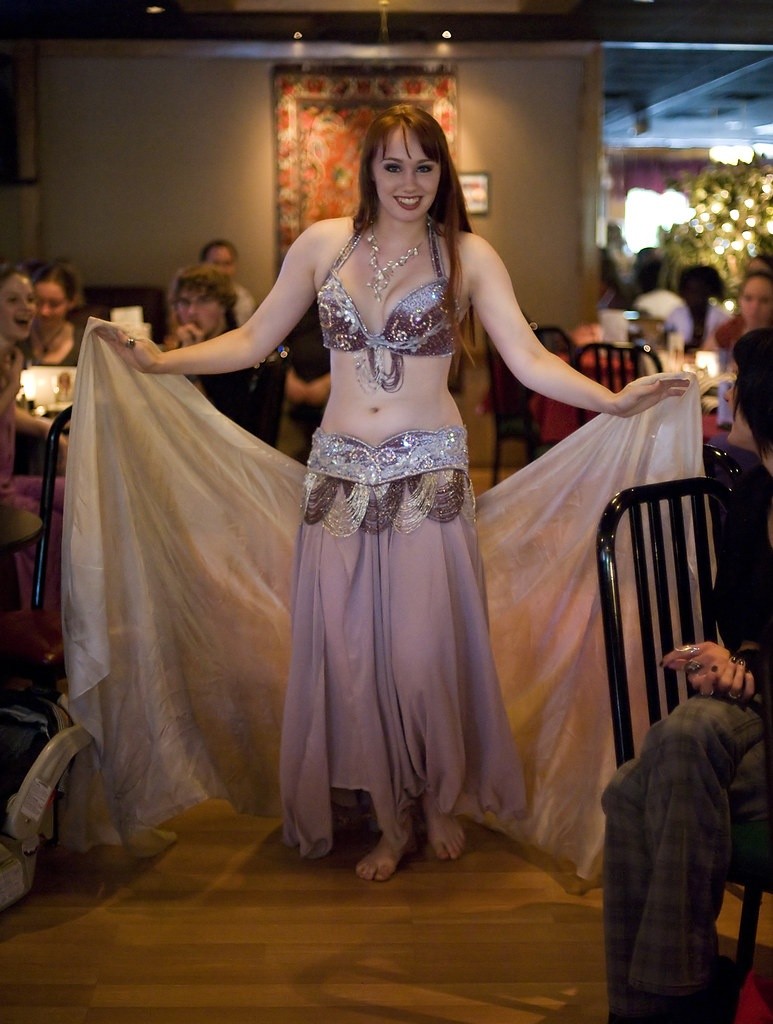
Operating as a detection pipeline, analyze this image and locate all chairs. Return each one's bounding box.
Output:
[1,398,75,693]
[237,352,288,449]
[480,322,772,989]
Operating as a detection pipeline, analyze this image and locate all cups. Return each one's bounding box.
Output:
[110,305,143,323]
[597,309,629,344]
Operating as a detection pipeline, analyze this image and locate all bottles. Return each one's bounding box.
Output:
[57,373,71,401]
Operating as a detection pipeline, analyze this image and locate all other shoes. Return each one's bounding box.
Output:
[656,955,740,1024]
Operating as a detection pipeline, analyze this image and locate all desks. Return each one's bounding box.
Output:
[524,346,732,439]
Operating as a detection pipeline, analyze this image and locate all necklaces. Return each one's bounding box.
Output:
[33,320,65,352]
[366,216,424,303]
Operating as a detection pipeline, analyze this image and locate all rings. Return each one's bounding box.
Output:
[125,338,137,349]
[728,690,742,699]
[672,644,701,656]
[700,689,713,698]
[682,658,702,674]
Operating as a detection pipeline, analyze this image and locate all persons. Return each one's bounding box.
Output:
[601,221,772,353]
[599,326,773,1024]
[161,239,331,450]
[89,102,691,882]
[0,265,68,664]
[16,261,100,369]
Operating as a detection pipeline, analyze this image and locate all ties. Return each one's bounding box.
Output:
[223,305,239,330]
